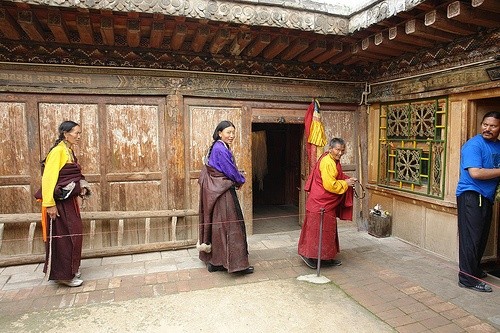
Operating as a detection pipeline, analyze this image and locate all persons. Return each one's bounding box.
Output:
[196,120,254,274]
[34,121,92,287]
[456,111,500,292]
[298,138,358,268]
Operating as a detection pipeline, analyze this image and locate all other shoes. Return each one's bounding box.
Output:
[458,279,493,293]
[55,271,84,287]
[207,264,254,276]
[479,271,488,278]
[301,255,343,269]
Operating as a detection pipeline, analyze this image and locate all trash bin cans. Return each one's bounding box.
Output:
[368,204,392,238]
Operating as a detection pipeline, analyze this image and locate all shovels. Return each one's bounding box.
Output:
[356,144,369,232]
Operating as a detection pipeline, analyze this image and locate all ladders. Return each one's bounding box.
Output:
[0,209,199,268]
